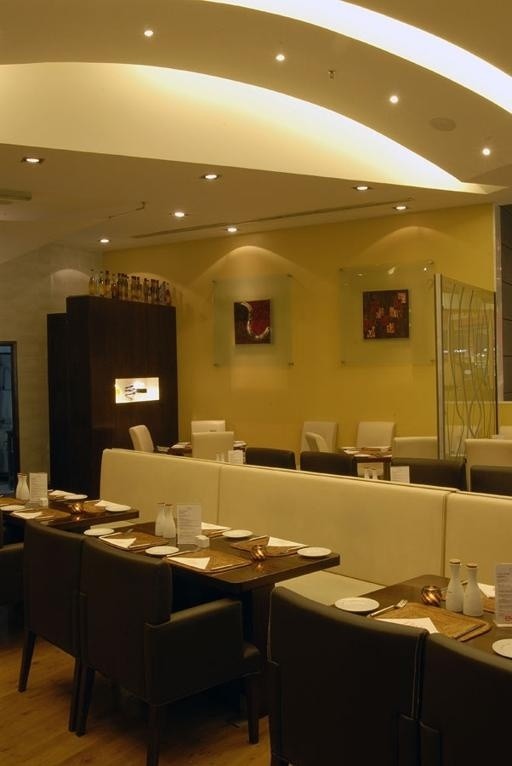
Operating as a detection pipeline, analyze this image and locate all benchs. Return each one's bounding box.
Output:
[422,633,512,766]
[219,465,457,608]
[100,449,220,526]
[268,586,429,766]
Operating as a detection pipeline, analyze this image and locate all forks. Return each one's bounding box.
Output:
[166,546,205,557]
[368,599,408,616]
[102,528,134,538]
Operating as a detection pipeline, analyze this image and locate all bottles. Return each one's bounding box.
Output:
[155,502,165,536]
[163,504,176,538]
[15,473,22,499]
[364,467,371,479]
[446,559,463,612]
[19,474,29,501]
[463,564,481,617]
[372,468,377,480]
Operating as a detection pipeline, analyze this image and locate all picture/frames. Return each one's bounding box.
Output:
[234,299,271,345]
[362,289,409,339]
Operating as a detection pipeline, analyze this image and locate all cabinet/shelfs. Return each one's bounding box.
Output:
[47,295,178,499]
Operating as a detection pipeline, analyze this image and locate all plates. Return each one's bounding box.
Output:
[335,597,380,612]
[146,545,180,556]
[222,530,253,537]
[65,494,89,500]
[297,546,333,558]
[104,503,131,512]
[85,527,115,537]
[1,505,26,510]
[491,638,512,659]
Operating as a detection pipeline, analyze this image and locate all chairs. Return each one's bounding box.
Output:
[356,421,395,451]
[391,457,467,493]
[18,521,97,733]
[191,420,226,433]
[303,421,339,452]
[300,452,357,476]
[77,538,260,766]
[446,493,512,585]
[393,437,438,458]
[302,432,328,453]
[0,544,23,642]
[129,425,184,456]
[470,465,512,496]
[351,457,390,481]
[191,432,233,459]
[246,448,296,470]
[464,439,512,492]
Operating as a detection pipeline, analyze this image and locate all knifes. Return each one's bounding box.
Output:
[213,561,249,572]
[233,533,268,546]
[449,622,485,641]
[128,541,166,547]
